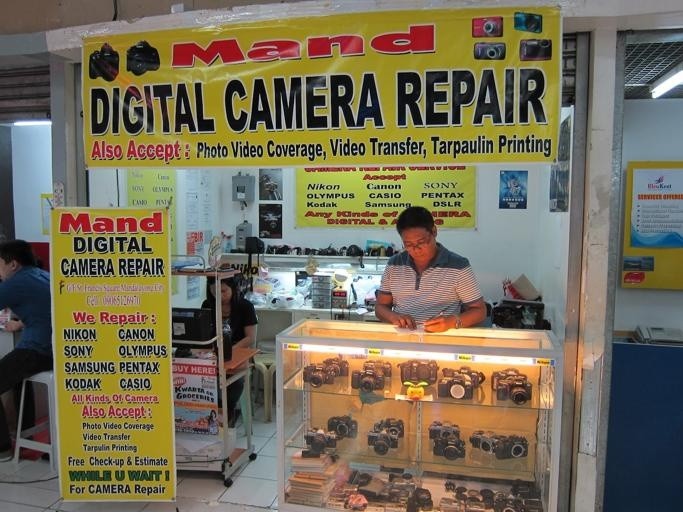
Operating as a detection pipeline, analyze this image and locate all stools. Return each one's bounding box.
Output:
[10,370,59,474]
[238,337,278,437]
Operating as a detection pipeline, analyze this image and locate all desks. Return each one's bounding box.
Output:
[171,255,262,486]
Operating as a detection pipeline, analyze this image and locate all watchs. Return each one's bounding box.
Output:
[452,313,461,329]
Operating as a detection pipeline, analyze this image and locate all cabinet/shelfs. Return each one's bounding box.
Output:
[214,253,395,316]
[272,317,562,512]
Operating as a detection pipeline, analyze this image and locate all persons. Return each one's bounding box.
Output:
[374,205,487,333]
[199,275,258,426]
[0,236,51,461]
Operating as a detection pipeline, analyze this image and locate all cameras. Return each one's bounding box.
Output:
[302,414,528,460]
[473,43,506,60]
[348,465,543,512]
[302,355,532,405]
[513,12,542,34]
[471,17,503,38]
[88,43,119,82]
[126,41,160,77]
[519,39,551,62]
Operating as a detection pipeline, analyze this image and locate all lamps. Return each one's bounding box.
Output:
[647,61,682,100]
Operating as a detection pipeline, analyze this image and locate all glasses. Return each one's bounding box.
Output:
[401,230,432,251]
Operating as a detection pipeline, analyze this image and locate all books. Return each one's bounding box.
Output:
[506,273,539,302]
[285,450,350,509]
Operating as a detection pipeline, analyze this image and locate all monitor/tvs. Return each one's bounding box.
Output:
[171,306,215,358]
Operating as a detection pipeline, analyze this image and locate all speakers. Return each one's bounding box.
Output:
[215,333,231,362]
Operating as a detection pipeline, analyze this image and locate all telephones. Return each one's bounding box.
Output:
[634,323,680,344]
